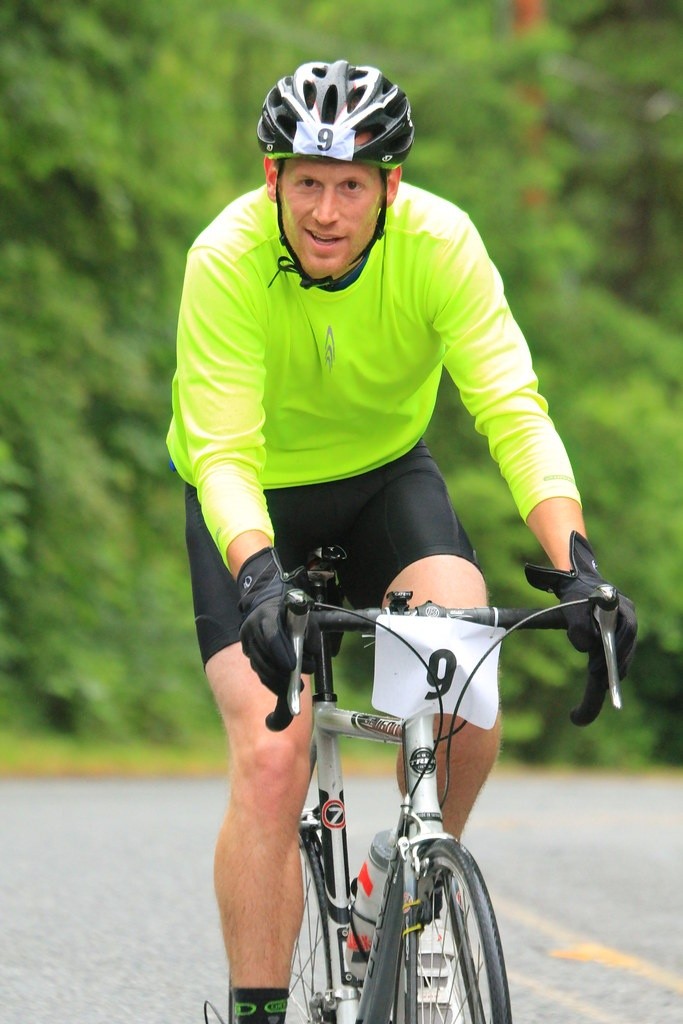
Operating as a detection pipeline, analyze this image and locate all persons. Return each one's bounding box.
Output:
[164,57,640,1024]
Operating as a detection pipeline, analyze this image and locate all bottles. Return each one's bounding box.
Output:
[343,827,398,981]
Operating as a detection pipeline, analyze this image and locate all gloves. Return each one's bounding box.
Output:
[235,546,322,695]
[524,529,638,728]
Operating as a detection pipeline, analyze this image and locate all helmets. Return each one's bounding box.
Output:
[256,59,415,170]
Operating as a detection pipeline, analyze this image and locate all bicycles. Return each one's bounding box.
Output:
[204,546,623,1024]
